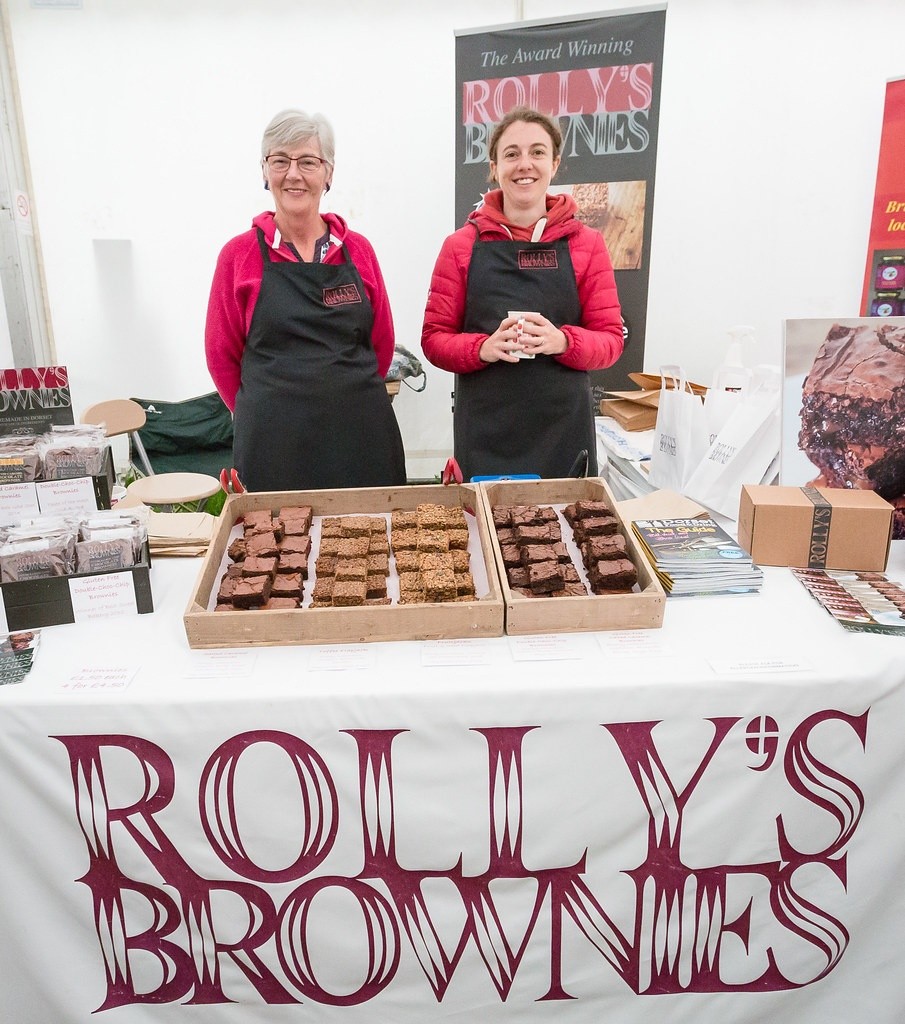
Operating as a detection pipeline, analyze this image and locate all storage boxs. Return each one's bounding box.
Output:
[0,467,43,516]
[183,482,504,648]
[0,506,153,633]
[737,485,895,577]
[35,445,116,513]
[478,476,665,638]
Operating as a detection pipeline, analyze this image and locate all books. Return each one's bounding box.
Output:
[631,510,763,596]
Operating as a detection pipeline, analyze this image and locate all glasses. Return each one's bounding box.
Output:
[263,155,333,173]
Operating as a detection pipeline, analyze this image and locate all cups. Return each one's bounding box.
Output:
[506,311,541,360]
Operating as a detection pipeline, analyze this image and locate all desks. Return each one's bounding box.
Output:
[0,510,905,1024]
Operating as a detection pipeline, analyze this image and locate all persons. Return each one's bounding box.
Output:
[420,105,624,482]
[204,110,407,493]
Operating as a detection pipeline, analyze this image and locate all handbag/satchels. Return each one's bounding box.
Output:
[648,364,783,521]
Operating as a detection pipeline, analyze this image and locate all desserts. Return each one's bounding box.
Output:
[215,504,479,611]
[493,500,639,599]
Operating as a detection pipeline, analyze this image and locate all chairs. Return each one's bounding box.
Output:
[79,400,220,513]
